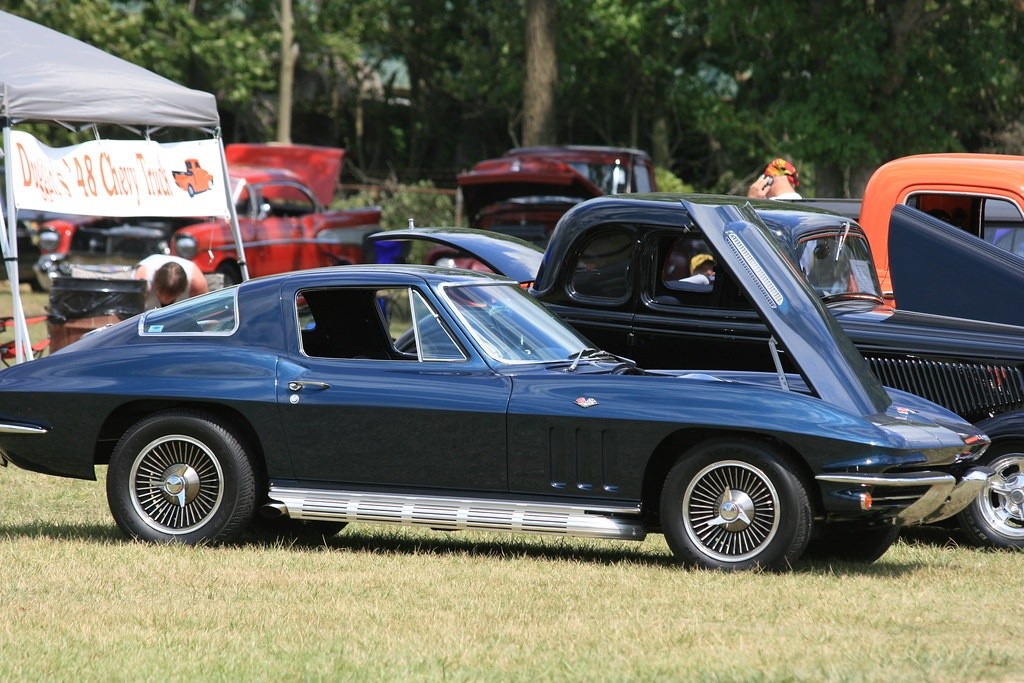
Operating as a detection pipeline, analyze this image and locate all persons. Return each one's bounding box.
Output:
[134,252,210,309]
[690,156,860,305]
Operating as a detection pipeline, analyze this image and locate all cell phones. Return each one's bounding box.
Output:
[762,175,774,190]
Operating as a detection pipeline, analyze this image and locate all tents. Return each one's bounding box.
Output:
[0,8,252,366]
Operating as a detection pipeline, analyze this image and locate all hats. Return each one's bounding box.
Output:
[690,253,716,273]
